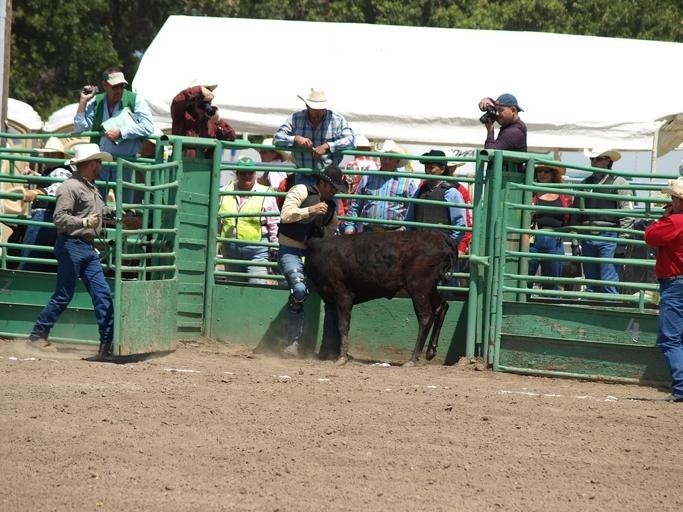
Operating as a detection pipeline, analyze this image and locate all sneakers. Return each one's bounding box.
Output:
[98,344,132,362]
[26,339,57,353]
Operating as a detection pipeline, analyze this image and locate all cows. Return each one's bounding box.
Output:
[301,195,459,368]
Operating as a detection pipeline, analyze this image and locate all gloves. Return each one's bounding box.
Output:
[269,248,279,261]
[571,244,581,256]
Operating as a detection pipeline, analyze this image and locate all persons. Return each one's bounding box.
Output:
[340,139,416,234]
[644,176,683,402]
[418,160,472,262]
[277,166,349,349]
[217,156,280,286]
[256,137,296,189]
[569,143,635,294]
[478,93,528,173]
[16,137,73,271]
[336,136,382,226]
[272,88,355,217]
[73,67,155,219]
[526,164,573,299]
[393,150,467,288]
[170,83,236,159]
[26,143,136,362]
[133,127,166,280]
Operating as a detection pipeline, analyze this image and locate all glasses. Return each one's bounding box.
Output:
[538,170,551,173]
[590,158,606,161]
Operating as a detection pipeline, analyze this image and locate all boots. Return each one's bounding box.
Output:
[288,296,304,340]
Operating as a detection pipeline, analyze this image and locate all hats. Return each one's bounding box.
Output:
[662,177,683,198]
[237,158,253,164]
[420,150,447,166]
[376,140,408,167]
[311,165,349,193]
[296,88,328,110]
[64,144,114,166]
[356,136,374,147]
[102,72,128,87]
[534,164,566,175]
[187,79,218,92]
[33,137,74,157]
[495,93,524,112]
[256,138,292,162]
[584,144,621,161]
[447,152,465,167]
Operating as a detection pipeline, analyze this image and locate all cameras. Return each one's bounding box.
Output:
[479,105,497,125]
[196,95,215,116]
